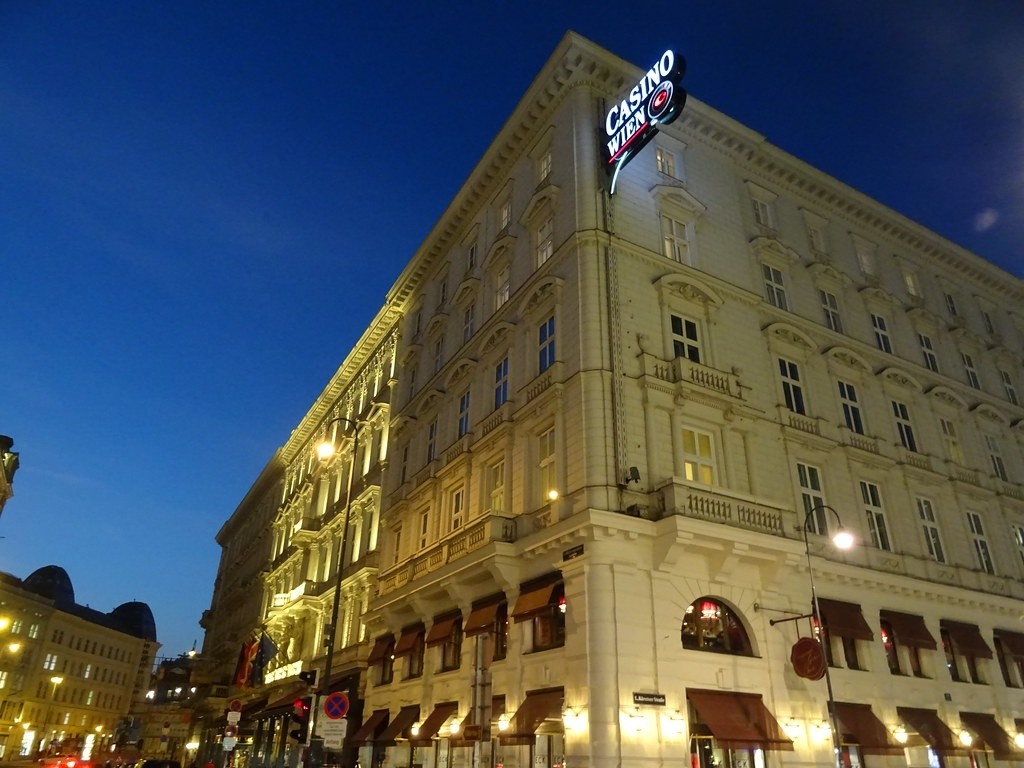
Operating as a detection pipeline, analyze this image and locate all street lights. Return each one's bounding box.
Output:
[803,505,854,768]
[313,418,359,696]
[31,676,63,762]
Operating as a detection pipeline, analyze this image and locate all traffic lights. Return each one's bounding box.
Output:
[290,696,311,744]
[300,669,316,686]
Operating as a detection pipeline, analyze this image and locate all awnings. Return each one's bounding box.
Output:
[368,568,564,666]
[348,686,564,746]
[827,700,1024,760]
[811,594,1024,667]
[685,687,796,752]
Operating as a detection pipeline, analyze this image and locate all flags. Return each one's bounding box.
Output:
[230,630,281,691]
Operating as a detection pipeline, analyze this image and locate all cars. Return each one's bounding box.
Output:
[135,759,182,768]
[38,754,67,765]
